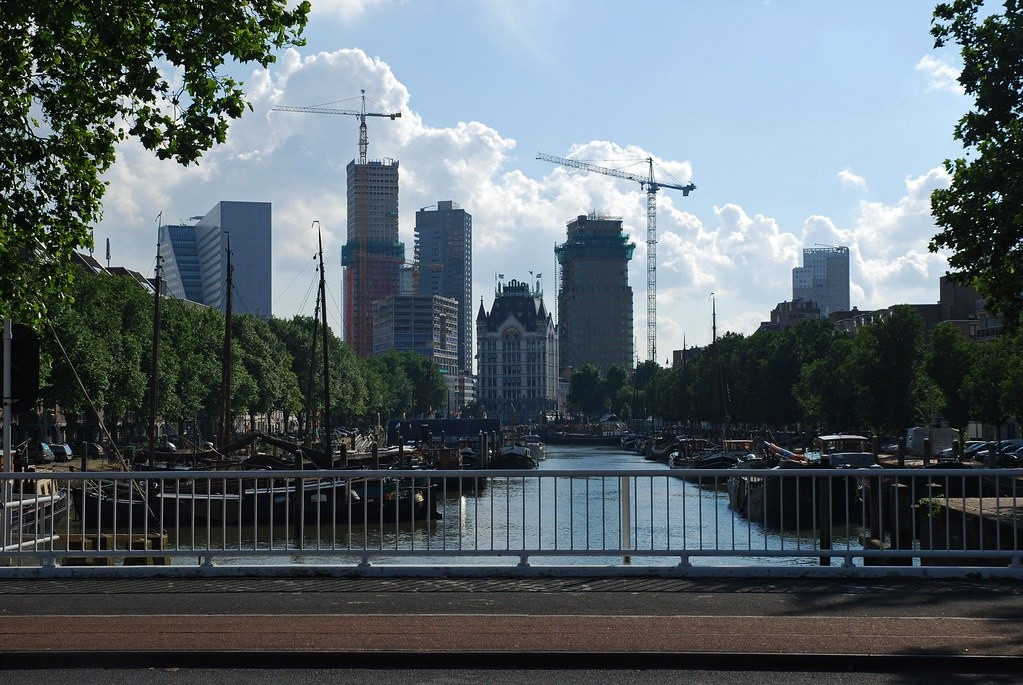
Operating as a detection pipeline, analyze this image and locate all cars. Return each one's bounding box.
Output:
[331,430,348,438]
[938,438,1023,468]
[882,441,900,455]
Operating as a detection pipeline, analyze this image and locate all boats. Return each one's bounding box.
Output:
[621,420,896,534]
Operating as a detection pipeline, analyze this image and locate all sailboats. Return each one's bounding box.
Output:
[0,224,546,566]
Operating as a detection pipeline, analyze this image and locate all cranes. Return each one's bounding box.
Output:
[271,88,402,163]
[535,150,698,363]
